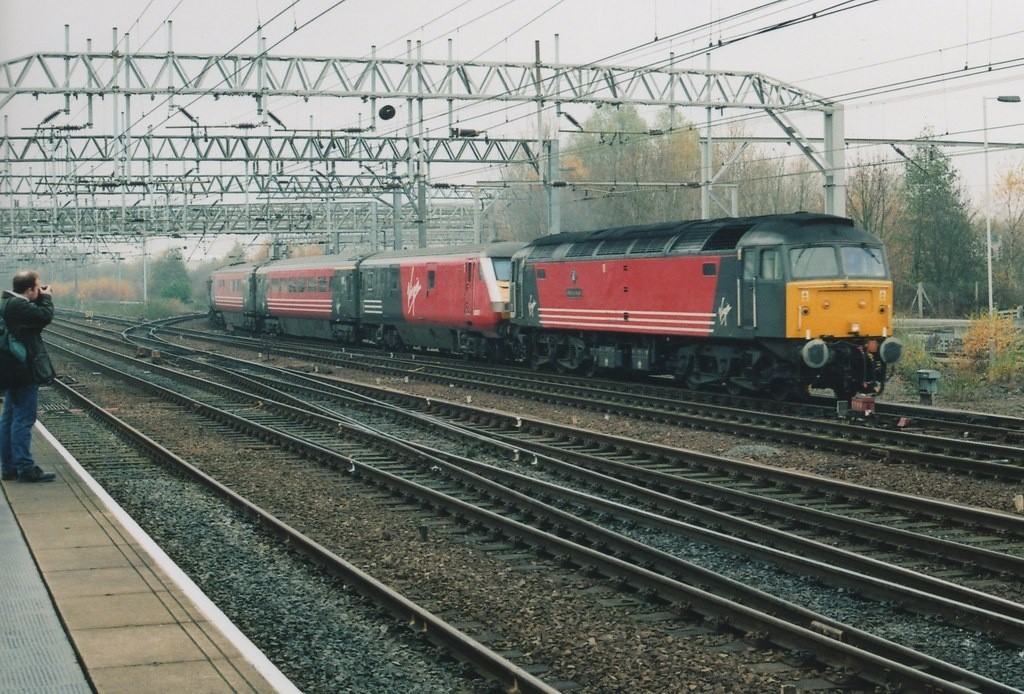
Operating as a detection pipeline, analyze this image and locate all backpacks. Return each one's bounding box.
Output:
[1,297,26,367]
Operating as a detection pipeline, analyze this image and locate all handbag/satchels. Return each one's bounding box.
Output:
[32,353,56,384]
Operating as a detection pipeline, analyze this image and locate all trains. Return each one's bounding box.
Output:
[203,212,906,401]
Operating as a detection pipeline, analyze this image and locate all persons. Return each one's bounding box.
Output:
[0,270,60,483]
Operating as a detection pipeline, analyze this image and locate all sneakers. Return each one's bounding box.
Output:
[18,466,55,482]
[2,473,17,480]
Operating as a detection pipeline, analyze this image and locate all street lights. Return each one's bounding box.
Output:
[981,94,1020,380]
